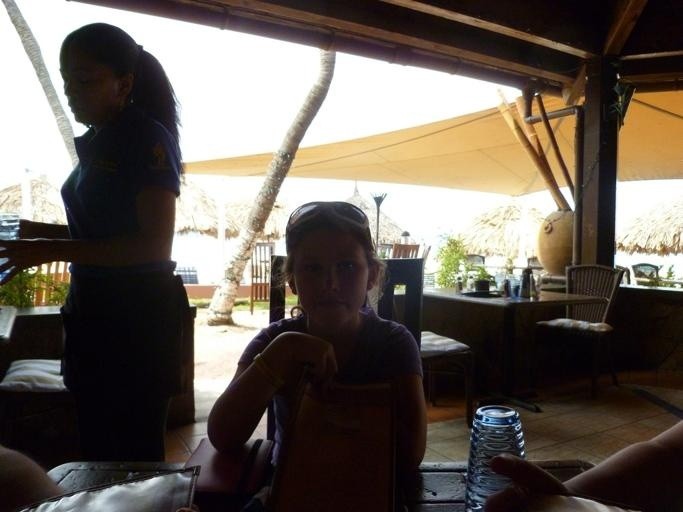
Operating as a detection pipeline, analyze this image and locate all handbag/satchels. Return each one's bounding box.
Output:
[185,436,273,496]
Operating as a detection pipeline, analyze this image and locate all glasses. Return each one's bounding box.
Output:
[282,202,369,233]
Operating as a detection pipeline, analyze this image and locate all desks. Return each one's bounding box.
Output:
[422,290,609,415]
[634,277,683,288]
[12,457,602,512]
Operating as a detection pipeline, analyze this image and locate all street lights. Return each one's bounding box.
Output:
[372,192,385,250]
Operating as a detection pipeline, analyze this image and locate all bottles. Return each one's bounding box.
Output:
[504,280,511,297]
[455,277,463,294]
[467,276,474,291]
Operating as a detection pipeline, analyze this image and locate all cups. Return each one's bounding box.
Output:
[529,274,541,301]
[0,213,20,280]
[507,274,523,300]
[475,280,489,290]
[466,405,526,510]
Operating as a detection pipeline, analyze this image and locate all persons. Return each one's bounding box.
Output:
[206,202,428,471]
[0,24,187,462]
[484,419,683,512]
[0,444,65,512]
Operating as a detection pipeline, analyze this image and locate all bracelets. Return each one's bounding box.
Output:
[253,354,285,390]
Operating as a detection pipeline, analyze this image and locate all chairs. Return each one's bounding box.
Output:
[392,244,420,258]
[0,348,75,450]
[377,257,474,428]
[422,246,431,262]
[616,265,631,287]
[633,264,659,279]
[528,265,630,399]
